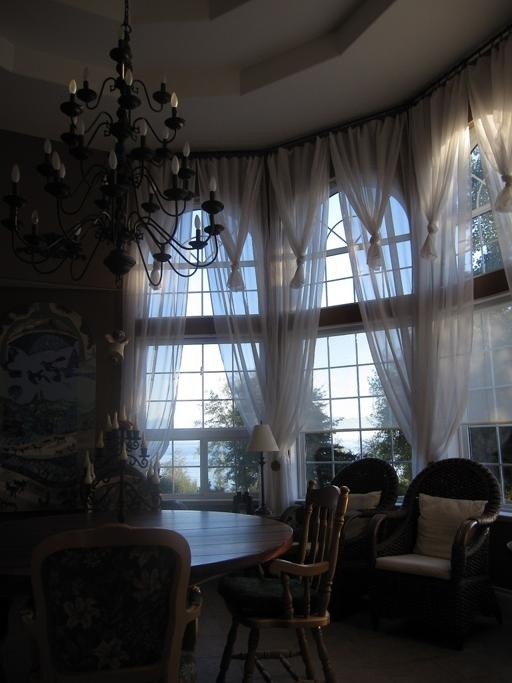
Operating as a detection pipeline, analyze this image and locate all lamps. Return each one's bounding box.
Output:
[0,0,225,289]
[79,405,160,523]
[245,421,279,514]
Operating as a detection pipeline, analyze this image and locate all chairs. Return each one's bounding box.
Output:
[210,480,350,683]
[21,523,204,683]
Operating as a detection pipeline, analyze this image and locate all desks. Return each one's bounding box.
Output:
[0,509,294,586]
